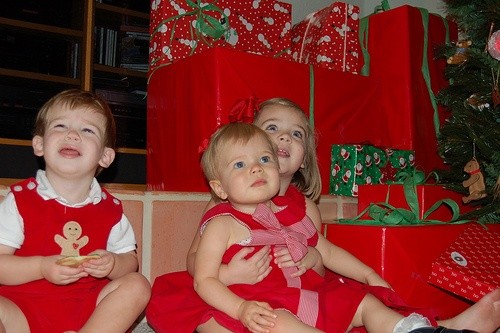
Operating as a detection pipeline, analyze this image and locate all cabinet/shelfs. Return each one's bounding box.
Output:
[0,0,149,192]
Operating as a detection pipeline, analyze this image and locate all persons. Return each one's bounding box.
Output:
[146,98,500,332]
[191,122,438,333]
[1,90,151,333]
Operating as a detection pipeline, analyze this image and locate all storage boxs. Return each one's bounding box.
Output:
[150,0,500,329]
